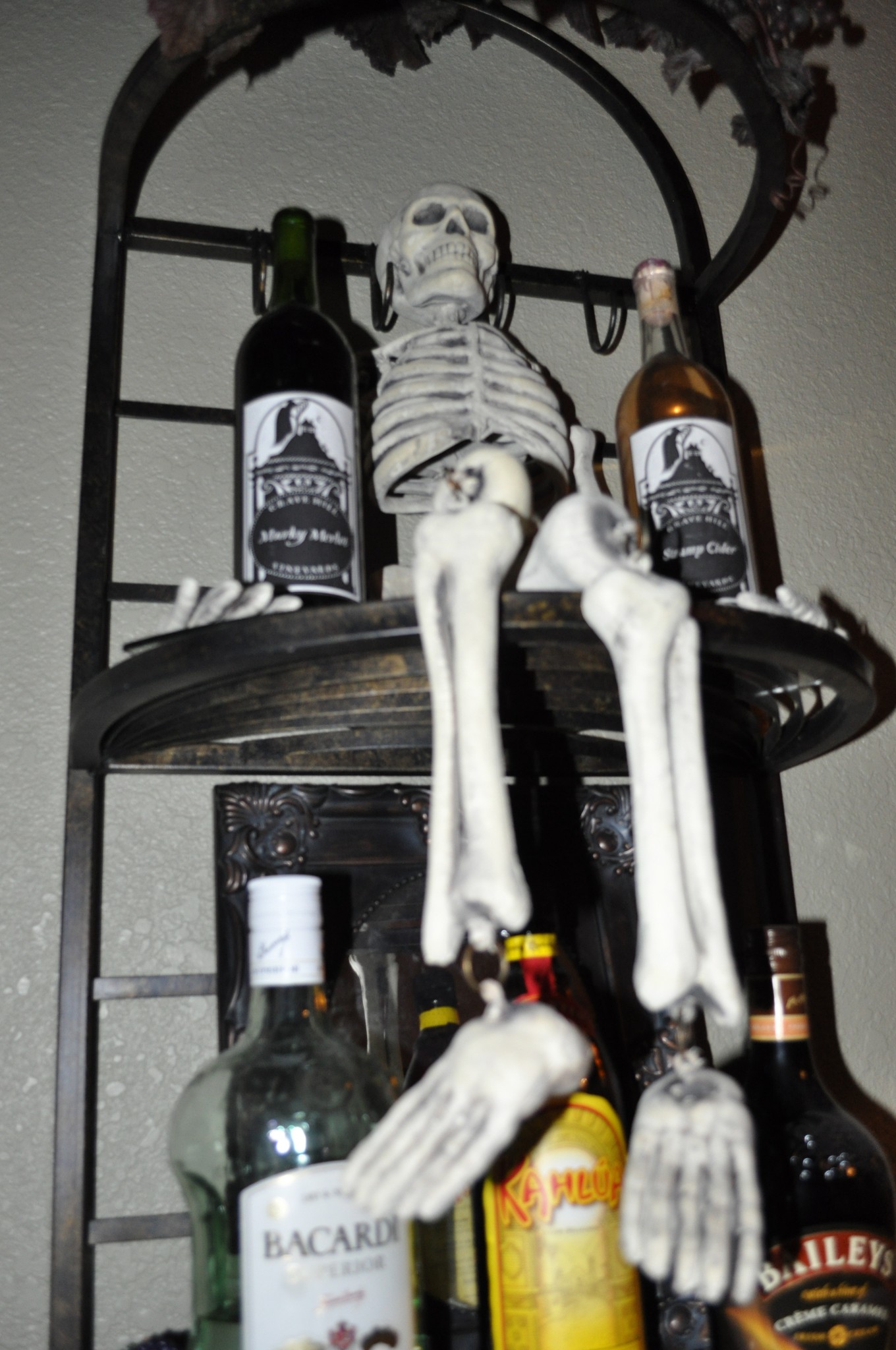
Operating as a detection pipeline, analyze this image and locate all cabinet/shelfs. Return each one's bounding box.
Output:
[47,0,896,1350]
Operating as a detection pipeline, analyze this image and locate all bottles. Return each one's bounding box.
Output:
[233,205,367,613]
[703,925,896,1350]
[398,925,644,1350]
[166,874,416,1350]
[613,258,757,608]
